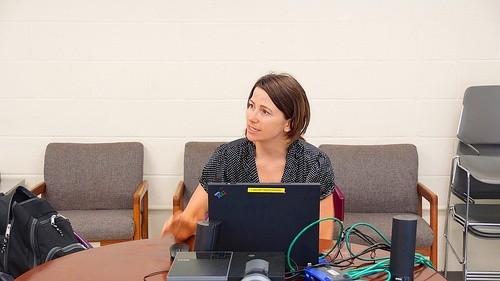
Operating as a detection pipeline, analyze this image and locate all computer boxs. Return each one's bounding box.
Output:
[166,252,286,281]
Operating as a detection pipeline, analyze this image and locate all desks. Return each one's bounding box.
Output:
[14,235,448,281]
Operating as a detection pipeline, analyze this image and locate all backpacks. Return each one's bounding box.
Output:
[0,186,87,281]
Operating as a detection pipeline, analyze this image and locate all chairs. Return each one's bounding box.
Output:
[173,142,225,252]
[320,143,439,273]
[444,85,500,281]
[29,142,149,246]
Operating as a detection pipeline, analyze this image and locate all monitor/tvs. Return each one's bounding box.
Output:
[206,182,320,270]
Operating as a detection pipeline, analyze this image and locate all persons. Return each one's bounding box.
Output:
[160,73,334,240]
